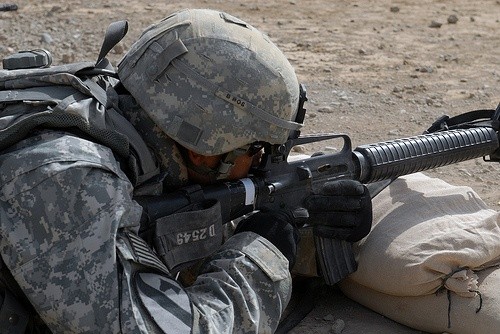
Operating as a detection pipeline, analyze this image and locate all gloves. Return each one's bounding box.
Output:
[234,205,309,271]
[306,150,372,241]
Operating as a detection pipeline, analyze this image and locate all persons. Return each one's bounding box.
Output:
[0,9,374,334]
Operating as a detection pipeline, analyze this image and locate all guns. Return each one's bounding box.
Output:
[133,83,500,287]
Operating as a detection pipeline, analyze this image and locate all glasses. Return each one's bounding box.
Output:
[235,141,266,158]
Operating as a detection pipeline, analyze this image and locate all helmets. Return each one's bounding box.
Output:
[115,9,307,185]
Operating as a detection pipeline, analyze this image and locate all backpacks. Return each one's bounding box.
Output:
[0,19,165,196]
